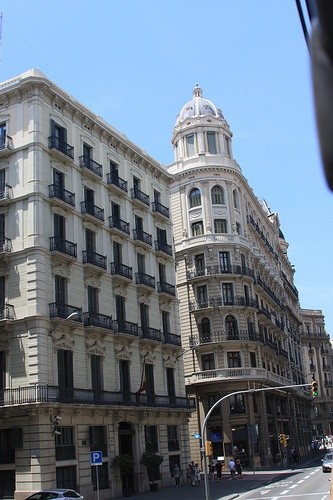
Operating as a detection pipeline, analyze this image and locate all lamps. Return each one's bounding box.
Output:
[175,344,200,361]
[50,312,79,336]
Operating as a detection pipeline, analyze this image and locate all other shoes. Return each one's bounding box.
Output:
[176,483,180,488]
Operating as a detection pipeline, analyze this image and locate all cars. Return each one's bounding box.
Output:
[321,453,333,473]
[25,489,83,500]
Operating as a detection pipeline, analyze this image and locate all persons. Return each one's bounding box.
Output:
[321,434,333,446]
[173,463,182,487]
[292,446,300,464]
[229,459,242,479]
[210,461,222,480]
[187,461,202,486]
[314,441,319,453]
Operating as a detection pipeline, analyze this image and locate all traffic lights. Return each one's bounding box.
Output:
[284,438,288,447]
[204,441,212,457]
[311,381,319,399]
[278,436,281,441]
[280,434,285,444]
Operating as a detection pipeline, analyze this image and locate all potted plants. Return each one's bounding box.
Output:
[111,453,137,497]
[139,452,164,492]
